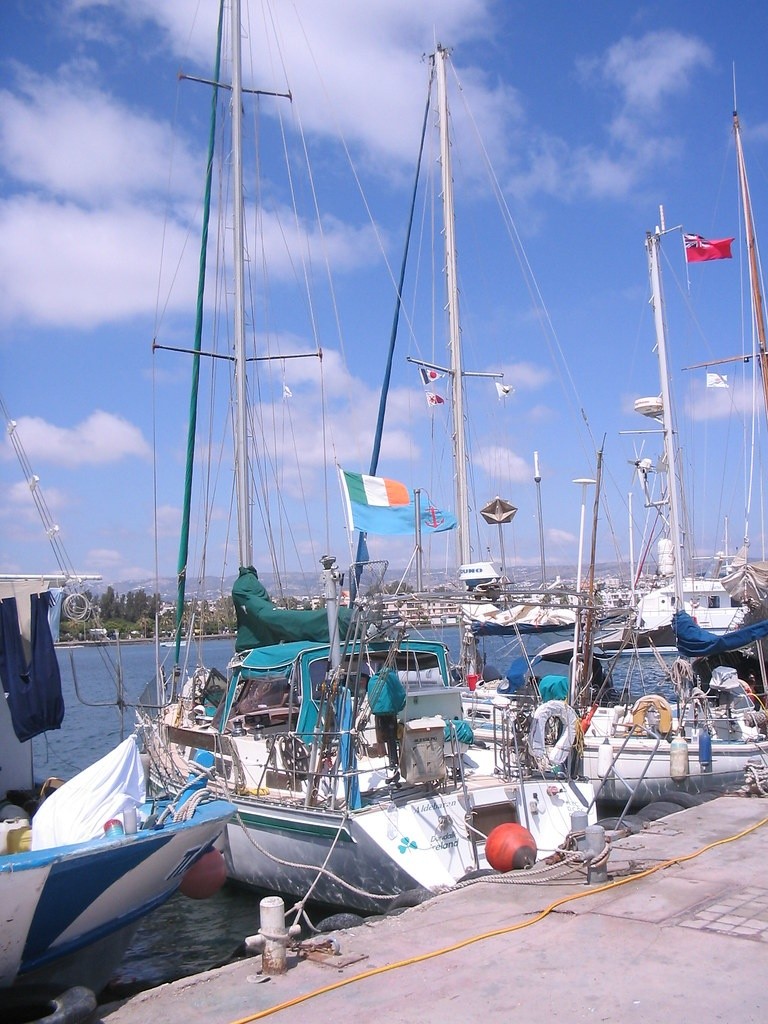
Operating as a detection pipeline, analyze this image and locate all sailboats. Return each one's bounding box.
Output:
[1,1,768,1000]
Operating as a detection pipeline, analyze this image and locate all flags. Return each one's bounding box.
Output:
[342,465,458,536]
[706,372,729,388]
[495,381,514,400]
[681,233,736,264]
[425,392,445,406]
[419,368,447,386]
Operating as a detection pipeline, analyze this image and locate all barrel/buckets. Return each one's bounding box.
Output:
[466,674,480,691]
[7,826,31,852]
[0,818,29,854]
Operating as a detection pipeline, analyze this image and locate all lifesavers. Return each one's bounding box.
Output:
[631,695,673,735]
[529,699,579,774]
